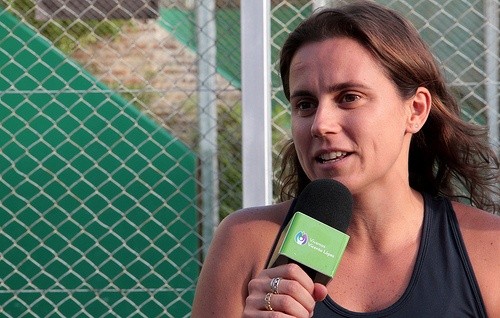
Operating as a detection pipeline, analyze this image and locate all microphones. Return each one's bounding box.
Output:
[265,178,353,287]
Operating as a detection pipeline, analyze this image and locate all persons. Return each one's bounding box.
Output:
[190,1,500,318]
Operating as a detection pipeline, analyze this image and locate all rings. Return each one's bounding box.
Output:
[263,292,274,311]
[270,276,283,293]
